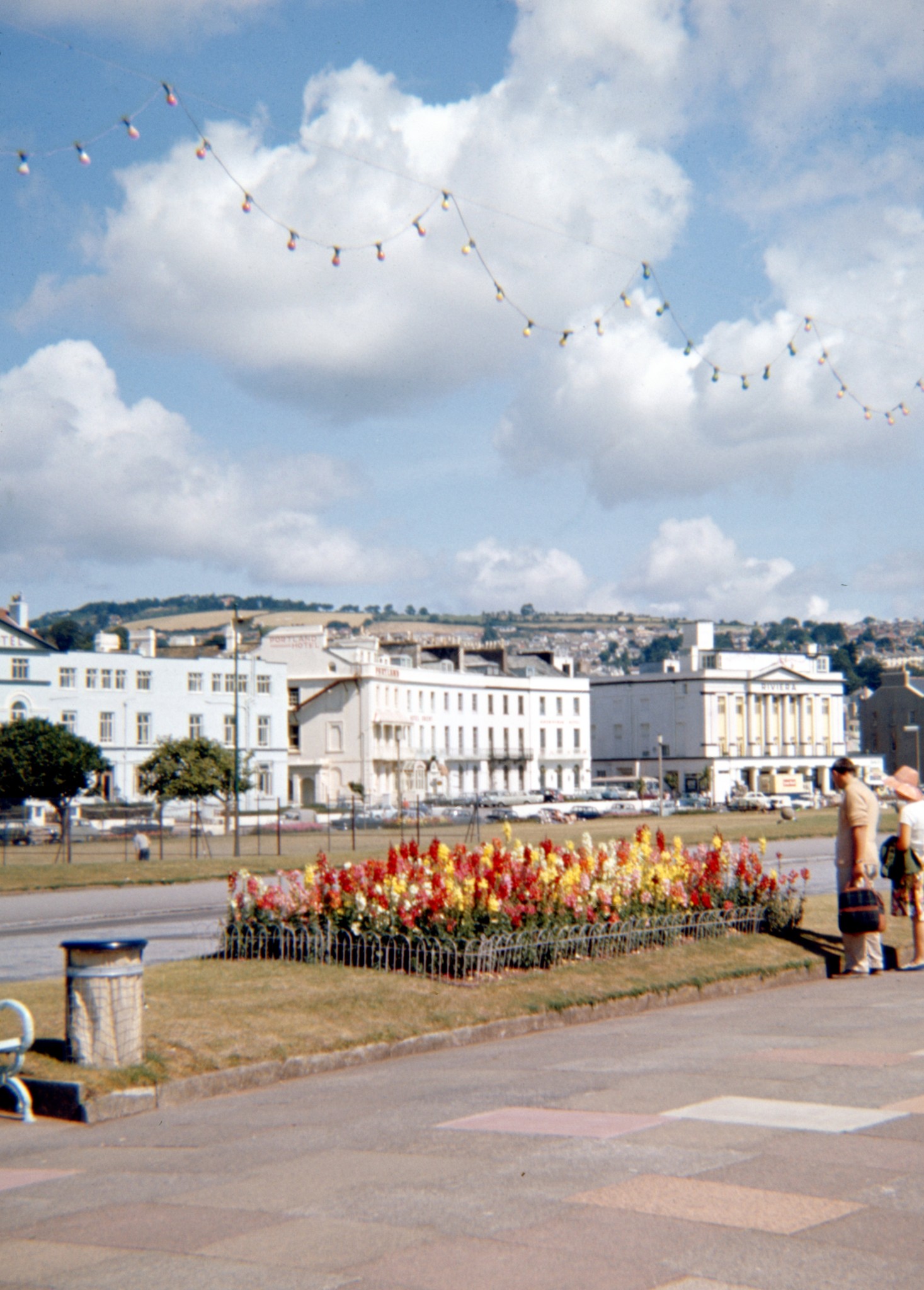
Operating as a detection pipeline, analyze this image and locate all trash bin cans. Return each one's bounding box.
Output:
[60,937,148,1069]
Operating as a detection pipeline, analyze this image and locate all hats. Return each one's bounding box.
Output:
[881,765,924,801]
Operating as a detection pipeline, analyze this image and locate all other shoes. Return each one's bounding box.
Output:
[831,969,868,978]
[869,968,883,975]
[897,960,924,971]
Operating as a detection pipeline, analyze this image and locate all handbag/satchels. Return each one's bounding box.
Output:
[838,872,886,935]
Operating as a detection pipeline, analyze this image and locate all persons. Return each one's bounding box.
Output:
[884,765,924,972]
[134,828,151,861]
[829,758,884,980]
